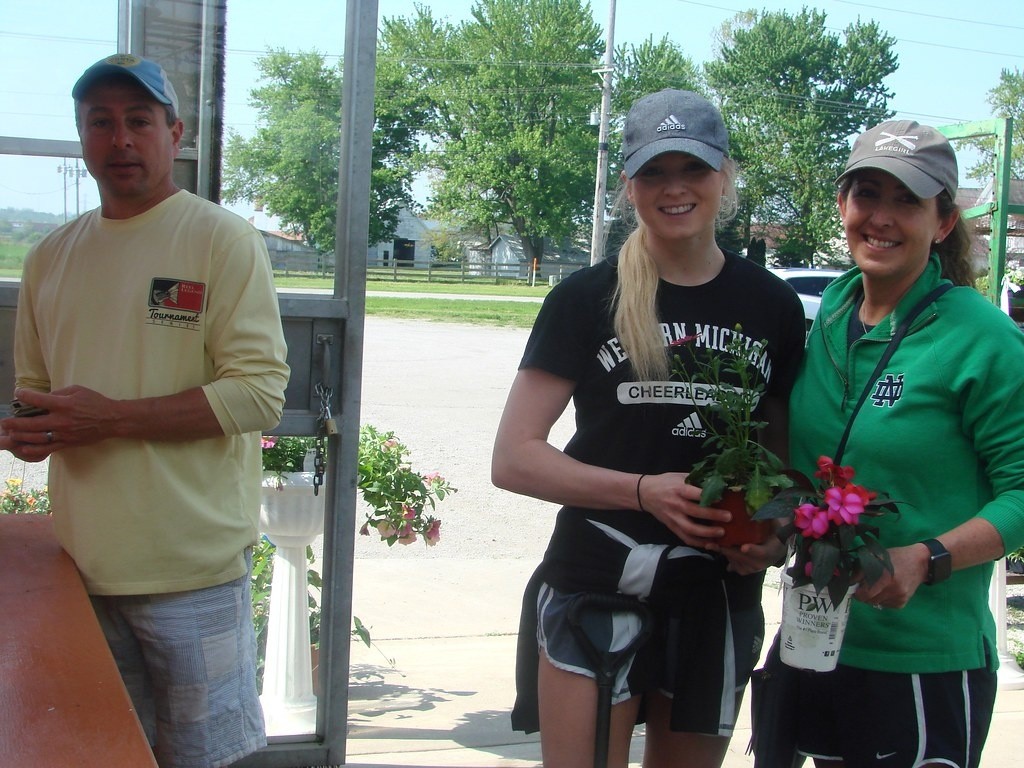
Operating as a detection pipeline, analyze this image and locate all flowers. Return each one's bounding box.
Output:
[668,321,795,516]
[260,434,457,547]
[750,455,919,613]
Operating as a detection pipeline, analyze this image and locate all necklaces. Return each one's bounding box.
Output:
[861,302,868,335]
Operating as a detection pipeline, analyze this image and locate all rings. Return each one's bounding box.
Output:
[47,431,53,443]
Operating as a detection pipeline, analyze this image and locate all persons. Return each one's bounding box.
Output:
[764,119,1022,768]
[491,89,806,768]
[0,53,289,768]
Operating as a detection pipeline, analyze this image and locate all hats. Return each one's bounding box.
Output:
[621,87,729,180]
[834,119,959,202]
[71,53,180,125]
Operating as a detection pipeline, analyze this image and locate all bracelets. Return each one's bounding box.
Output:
[636,474,648,512]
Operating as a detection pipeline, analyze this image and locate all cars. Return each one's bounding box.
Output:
[770,269,842,333]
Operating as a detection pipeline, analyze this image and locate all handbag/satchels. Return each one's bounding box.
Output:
[752,664,806,768]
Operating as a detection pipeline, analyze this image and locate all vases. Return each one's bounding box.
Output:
[717,487,769,550]
[260,470,317,736]
[780,569,859,672]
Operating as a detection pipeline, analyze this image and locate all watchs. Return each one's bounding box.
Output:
[919,539,952,586]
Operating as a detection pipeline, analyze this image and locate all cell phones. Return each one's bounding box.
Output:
[8,399,50,417]
